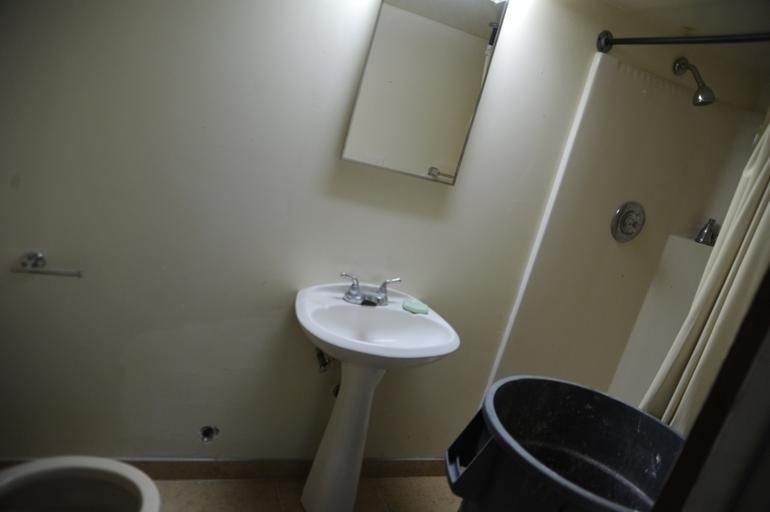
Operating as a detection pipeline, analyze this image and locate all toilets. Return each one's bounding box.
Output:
[0,454,162,512]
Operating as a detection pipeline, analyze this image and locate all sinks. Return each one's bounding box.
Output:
[295,282,461,369]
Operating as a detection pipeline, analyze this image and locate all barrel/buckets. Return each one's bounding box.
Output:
[444,375,681,512]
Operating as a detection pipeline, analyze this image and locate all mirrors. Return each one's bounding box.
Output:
[341,1,507,184]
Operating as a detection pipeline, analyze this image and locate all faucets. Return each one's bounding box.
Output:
[360,292,380,305]
